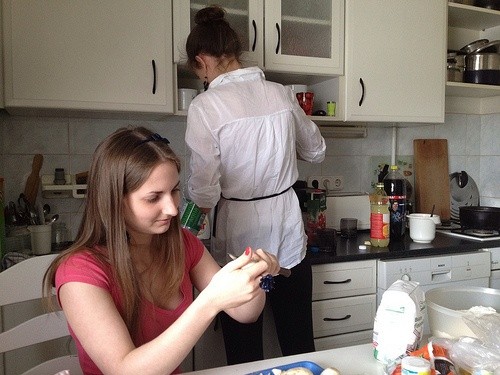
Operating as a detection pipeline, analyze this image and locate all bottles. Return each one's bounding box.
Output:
[370,182,390,248]
[179,199,206,236]
[54,168,66,185]
[384,166,408,241]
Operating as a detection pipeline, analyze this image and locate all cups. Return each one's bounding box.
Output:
[296,91,313,116]
[318,228,338,253]
[340,218,358,238]
[178,88,197,111]
[327,101,335,116]
[29,225,52,255]
[406,213,441,244]
[51,222,66,251]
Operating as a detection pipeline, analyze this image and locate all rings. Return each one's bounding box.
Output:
[258,274,275,292]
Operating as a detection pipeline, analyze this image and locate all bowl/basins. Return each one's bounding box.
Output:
[424,285,500,338]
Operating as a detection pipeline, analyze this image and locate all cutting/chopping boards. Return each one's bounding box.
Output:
[413,138,451,220]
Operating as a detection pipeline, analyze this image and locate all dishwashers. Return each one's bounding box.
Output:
[377,251,491,314]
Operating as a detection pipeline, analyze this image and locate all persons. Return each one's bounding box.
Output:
[390,200,402,226]
[186,6,326,365]
[43,126,280,375]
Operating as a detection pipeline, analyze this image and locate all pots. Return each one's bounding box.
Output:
[459,206,500,231]
[447,38,500,87]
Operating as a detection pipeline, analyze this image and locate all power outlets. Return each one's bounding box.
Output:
[321,176,332,190]
[332,176,344,191]
[307,176,321,189]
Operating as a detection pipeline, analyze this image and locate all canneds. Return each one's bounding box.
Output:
[401,356,431,375]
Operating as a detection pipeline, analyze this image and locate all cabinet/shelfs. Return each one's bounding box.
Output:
[0,0,500,128]
[193,260,377,373]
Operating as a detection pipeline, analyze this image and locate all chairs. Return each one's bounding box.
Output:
[0,254,84,375]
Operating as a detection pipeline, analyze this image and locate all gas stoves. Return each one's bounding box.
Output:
[436,220,500,271]
